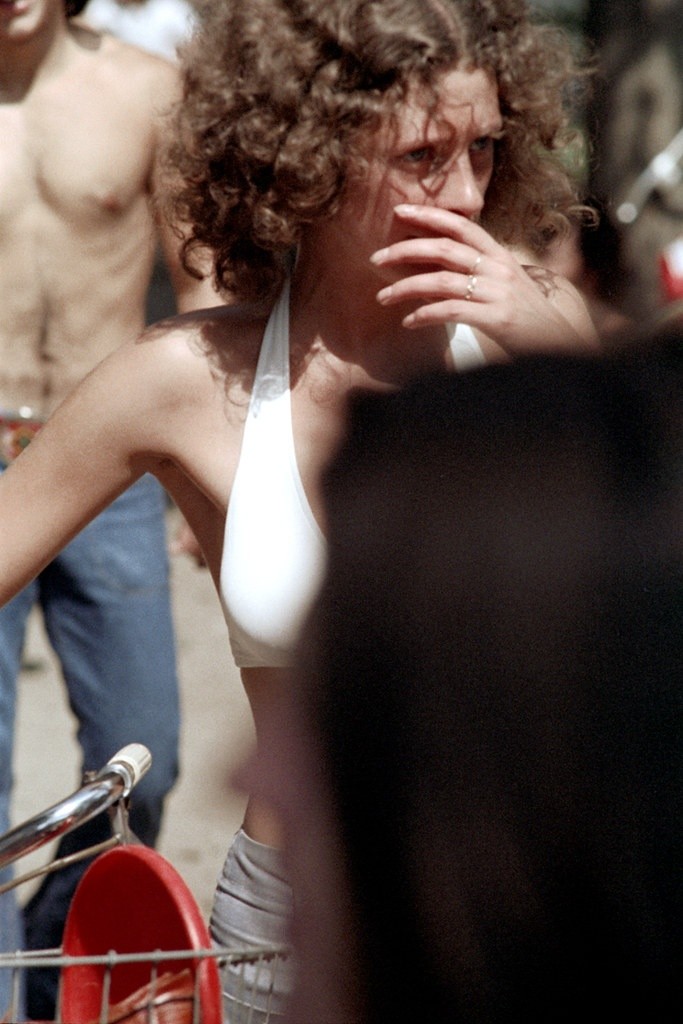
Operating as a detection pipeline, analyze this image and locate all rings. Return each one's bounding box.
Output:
[466,278,477,301]
[472,251,483,275]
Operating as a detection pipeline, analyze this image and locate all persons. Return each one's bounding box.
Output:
[0,0,604,1021]
[0,1,231,1024]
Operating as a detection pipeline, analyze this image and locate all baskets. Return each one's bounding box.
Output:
[0,944,293,1024]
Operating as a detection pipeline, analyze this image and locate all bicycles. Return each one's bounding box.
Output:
[0,743,293,1024]
